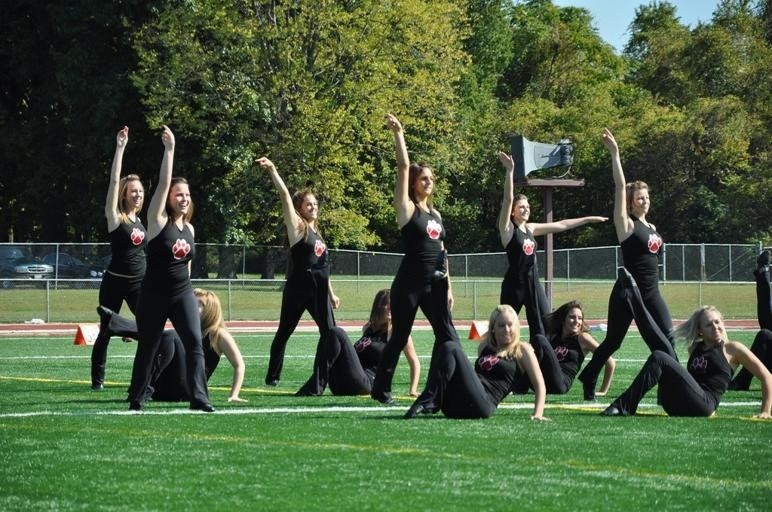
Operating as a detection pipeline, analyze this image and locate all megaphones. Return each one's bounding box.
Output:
[511,135,572,180]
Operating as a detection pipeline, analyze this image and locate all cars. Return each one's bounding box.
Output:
[0,247,107,289]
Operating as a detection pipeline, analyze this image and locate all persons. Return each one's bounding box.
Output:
[511,300,616,396]
[128,123,215,412]
[370,113,454,405]
[254,156,341,387]
[293,288,423,396]
[496,150,611,395]
[598,266,772,419]
[97,288,250,403]
[727,249,772,391]
[577,127,676,405]
[404,303,554,421]
[90,125,148,391]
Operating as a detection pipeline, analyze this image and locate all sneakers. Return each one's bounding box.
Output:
[189,401,216,412]
[91,383,103,391]
[371,392,396,405]
[265,379,281,388]
[726,379,751,391]
[401,400,434,419]
[523,252,537,278]
[583,382,598,402]
[96,305,114,336]
[294,390,316,397]
[431,249,448,280]
[599,405,621,416]
[752,250,770,277]
[129,402,144,411]
[617,266,638,301]
[306,248,330,274]
